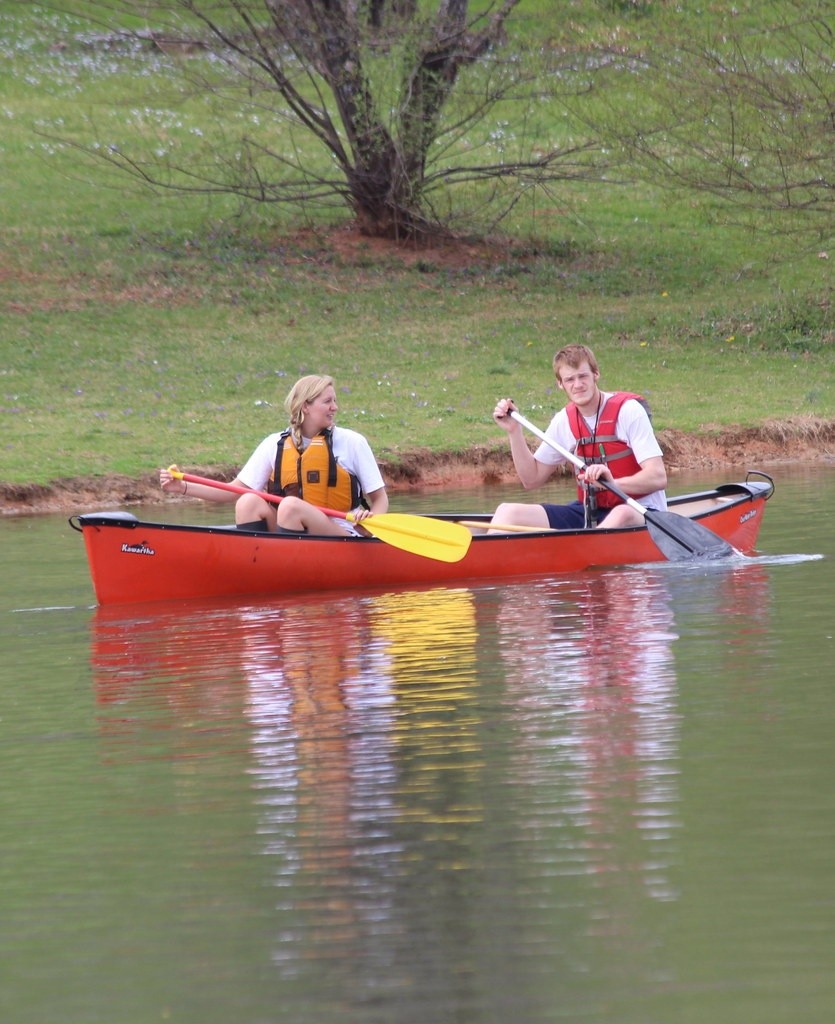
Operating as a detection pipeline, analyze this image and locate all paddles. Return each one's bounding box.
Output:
[165,465,473,564]
[500,397,734,560]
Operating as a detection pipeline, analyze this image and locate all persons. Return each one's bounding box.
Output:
[160,375,389,537]
[487,344,667,534]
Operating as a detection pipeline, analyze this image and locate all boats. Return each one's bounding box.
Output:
[65,469,778,612]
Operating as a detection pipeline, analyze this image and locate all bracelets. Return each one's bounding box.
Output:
[183,482,187,495]
